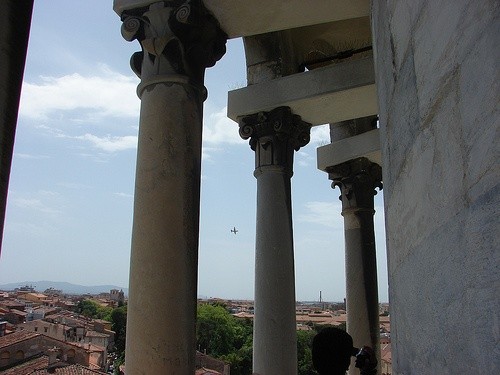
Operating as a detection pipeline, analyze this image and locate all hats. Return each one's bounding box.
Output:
[312,327,359,357]
[360,345,378,369]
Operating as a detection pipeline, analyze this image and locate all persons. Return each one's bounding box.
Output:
[355,345,378,375]
[310,327,359,375]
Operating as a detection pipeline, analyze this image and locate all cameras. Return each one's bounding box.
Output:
[351,346,369,369]
